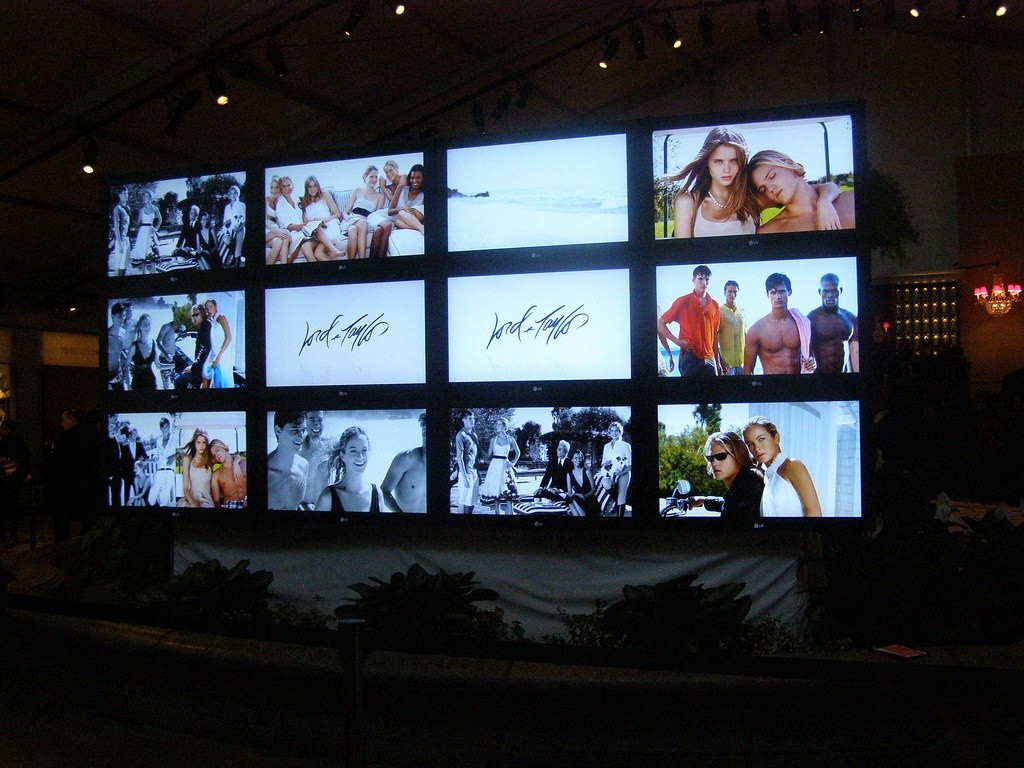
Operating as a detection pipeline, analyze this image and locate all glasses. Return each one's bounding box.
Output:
[192,313,200,318]
[705,452,734,462]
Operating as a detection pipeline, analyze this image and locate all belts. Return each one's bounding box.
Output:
[140,223,153,226]
[492,454,507,459]
[158,468,174,472]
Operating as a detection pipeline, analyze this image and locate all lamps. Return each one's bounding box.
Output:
[974,264,1024,320]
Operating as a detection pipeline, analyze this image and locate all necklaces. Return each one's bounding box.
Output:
[708,191,725,208]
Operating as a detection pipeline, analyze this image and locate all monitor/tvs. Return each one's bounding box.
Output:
[96,99,883,534]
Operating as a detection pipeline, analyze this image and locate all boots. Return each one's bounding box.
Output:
[617,504,625,518]
[226,239,235,266]
[234,256,241,268]
[606,486,619,514]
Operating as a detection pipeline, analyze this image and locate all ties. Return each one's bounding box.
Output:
[558,460,562,469]
[191,223,194,229]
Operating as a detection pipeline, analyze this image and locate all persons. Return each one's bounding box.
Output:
[742,273,858,375]
[455,410,520,513]
[747,150,855,234]
[50,409,99,538]
[342,166,383,259]
[109,414,247,507]
[265,175,291,264]
[108,302,177,390]
[266,409,427,513]
[0,419,28,546]
[130,191,162,275]
[174,205,221,270]
[742,416,822,517]
[369,160,424,259]
[223,185,246,268]
[276,177,346,262]
[174,298,231,389]
[533,421,632,517]
[114,187,132,276]
[687,430,764,518]
[656,265,747,376]
[301,177,342,262]
[664,126,841,237]
